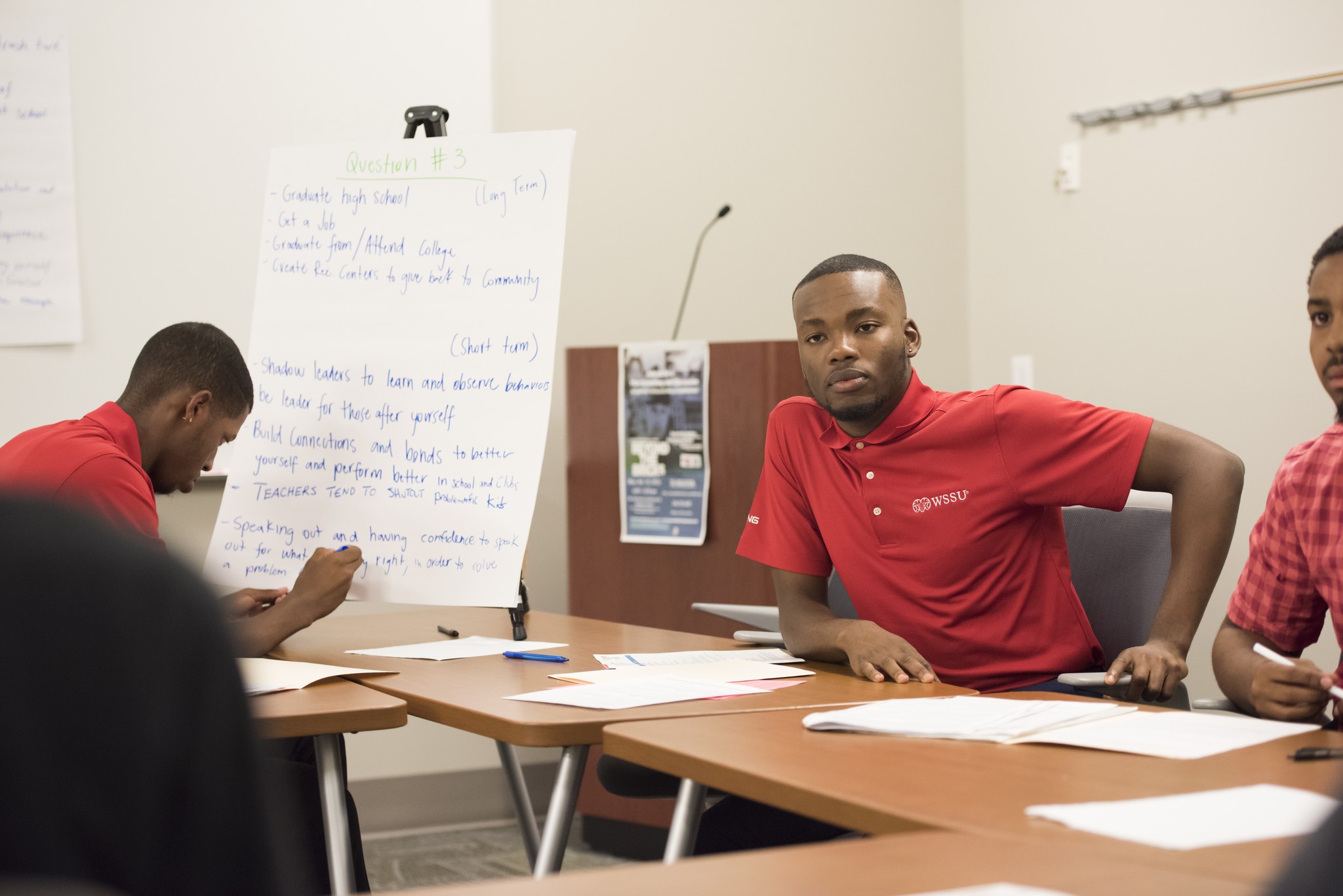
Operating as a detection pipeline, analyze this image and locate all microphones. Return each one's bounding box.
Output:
[671,205,730,343]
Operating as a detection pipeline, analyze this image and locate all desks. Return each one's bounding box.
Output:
[249,603,1343,896]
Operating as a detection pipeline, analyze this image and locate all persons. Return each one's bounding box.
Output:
[0,323,370,896]
[691,255,1245,857]
[1212,226,1343,731]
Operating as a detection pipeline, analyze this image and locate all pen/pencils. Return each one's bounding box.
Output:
[1252,642,1343,699]
[1285,746,1343,762]
[503,650,570,663]
[438,624,459,637]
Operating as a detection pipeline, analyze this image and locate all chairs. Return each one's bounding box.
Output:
[1062,508,1192,712]
